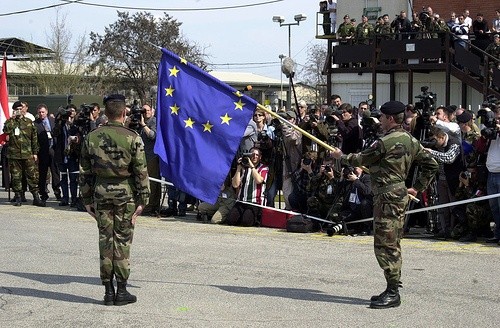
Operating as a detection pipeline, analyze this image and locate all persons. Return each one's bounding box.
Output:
[319,0,337,35]
[2,94,500,241]
[329,101,441,309]
[336,6,500,92]
[77,94,151,306]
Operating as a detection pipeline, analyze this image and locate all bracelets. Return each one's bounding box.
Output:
[251,166,255,169]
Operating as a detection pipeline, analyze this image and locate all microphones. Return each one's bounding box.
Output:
[281,59,294,76]
[362,110,372,118]
[324,108,333,117]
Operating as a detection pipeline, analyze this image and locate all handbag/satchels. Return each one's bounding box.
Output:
[286,215,321,233]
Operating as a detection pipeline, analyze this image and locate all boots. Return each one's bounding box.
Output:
[116,281,137,306]
[370,290,402,309]
[103,281,116,305]
[10,183,202,221]
[371,289,388,302]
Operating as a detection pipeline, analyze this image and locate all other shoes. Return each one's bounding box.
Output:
[319,216,500,245]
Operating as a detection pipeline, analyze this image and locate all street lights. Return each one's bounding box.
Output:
[272,14,307,109]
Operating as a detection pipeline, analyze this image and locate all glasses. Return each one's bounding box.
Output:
[253,113,264,116]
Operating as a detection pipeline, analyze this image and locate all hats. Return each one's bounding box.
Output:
[297,100,308,107]
[103,94,126,105]
[12,101,22,110]
[282,111,296,119]
[380,101,406,115]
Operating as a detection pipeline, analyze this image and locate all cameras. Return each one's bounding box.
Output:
[343,166,354,176]
[308,105,318,122]
[241,153,253,166]
[324,165,331,172]
[303,157,312,165]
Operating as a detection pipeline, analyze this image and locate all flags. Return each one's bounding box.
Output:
[153,48,257,205]
[0,59,11,147]
